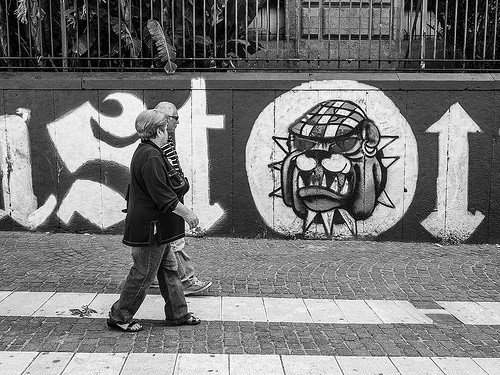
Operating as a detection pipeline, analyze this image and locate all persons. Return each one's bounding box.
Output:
[150,102,212,295]
[107,110,201,333]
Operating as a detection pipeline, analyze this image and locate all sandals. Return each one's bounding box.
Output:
[107,318,143,332]
[181,316,200,325]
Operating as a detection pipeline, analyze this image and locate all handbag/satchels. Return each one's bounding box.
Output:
[166,160,189,195]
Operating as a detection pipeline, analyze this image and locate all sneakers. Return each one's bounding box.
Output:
[183,280,212,295]
[149,282,159,288]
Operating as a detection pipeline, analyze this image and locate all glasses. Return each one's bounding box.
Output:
[169,116,178,120]
